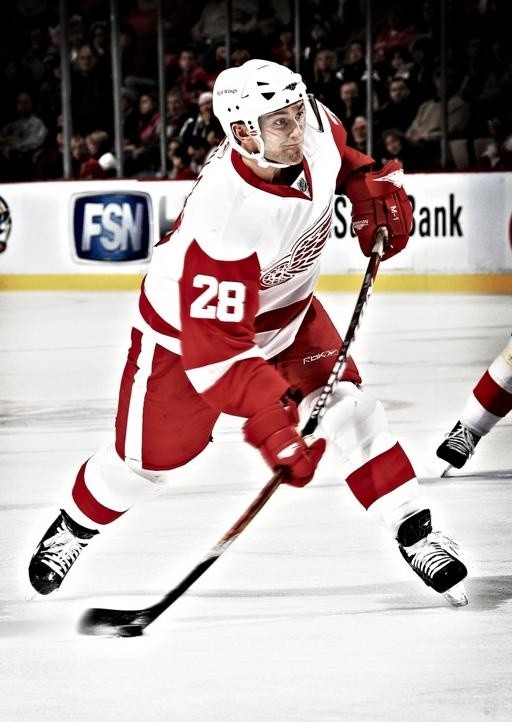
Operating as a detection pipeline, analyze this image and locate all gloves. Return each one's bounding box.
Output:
[241,402,327,488]
[346,158,414,262]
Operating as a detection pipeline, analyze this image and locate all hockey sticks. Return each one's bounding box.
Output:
[76,226,392,635]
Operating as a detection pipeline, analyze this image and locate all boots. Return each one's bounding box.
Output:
[28,508,101,596]
[436,419,481,470]
[394,508,469,594]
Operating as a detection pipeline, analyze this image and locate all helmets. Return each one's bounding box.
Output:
[211,59,309,169]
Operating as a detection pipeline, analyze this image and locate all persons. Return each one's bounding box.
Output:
[436,215,512,469]
[29,59,469,595]
